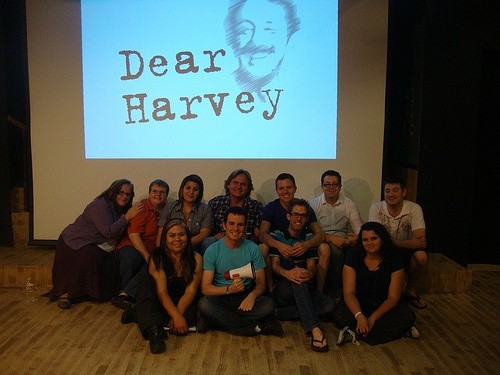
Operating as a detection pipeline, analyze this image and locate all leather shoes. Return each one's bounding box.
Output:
[197,312,210,331]
[264,319,288,337]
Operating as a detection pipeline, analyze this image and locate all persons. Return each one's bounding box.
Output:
[222,0,300,99]
[196,206,285,338]
[368,177,428,309]
[198,168,265,256]
[112,179,170,323]
[134,218,203,355]
[154,173,213,256]
[331,222,420,346]
[308,170,363,258]
[268,198,339,352]
[259,173,326,258]
[41,179,144,310]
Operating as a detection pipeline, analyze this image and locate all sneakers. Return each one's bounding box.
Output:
[407,326,420,339]
[335,327,355,345]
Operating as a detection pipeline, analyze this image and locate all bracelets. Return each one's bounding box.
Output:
[226,286,230,296]
[354,312,363,319]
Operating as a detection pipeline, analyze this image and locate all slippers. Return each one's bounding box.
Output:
[311,333,330,353]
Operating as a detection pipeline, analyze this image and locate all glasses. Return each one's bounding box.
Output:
[117,191,133,197]
[323,183,340,188]
[289,212,308,218]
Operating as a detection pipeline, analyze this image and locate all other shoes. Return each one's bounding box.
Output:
[58,296,72,308]
[146,326,167,353]
[113,293,131,309]
[406,293,426,309]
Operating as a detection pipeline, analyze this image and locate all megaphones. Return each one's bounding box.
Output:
[224,261,256,280]
